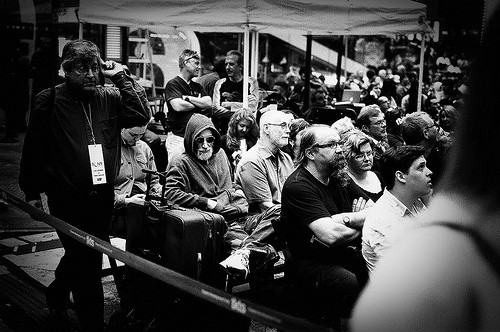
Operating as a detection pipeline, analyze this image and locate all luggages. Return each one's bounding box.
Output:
[142,169,228,306]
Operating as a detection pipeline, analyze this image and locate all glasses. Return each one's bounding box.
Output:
[185,55,199,60]
[76,64,100,73]
[355,151,373,161]
[315,140,344,148]
[268,122,291,127]
[194,136,215,143]
[425,124,438,129]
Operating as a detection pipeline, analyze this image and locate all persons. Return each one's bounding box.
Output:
[18,41,468,332]
[349,7,499,331]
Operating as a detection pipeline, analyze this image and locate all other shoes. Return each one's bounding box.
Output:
[45,291,69,323]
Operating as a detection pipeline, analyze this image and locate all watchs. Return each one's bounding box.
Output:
[340,213,351,227]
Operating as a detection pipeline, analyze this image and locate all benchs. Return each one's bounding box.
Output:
[101,215,350,332]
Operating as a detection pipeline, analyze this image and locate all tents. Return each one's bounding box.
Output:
[79,0,434,112]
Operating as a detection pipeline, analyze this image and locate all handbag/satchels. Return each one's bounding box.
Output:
[19,159,55,198]
